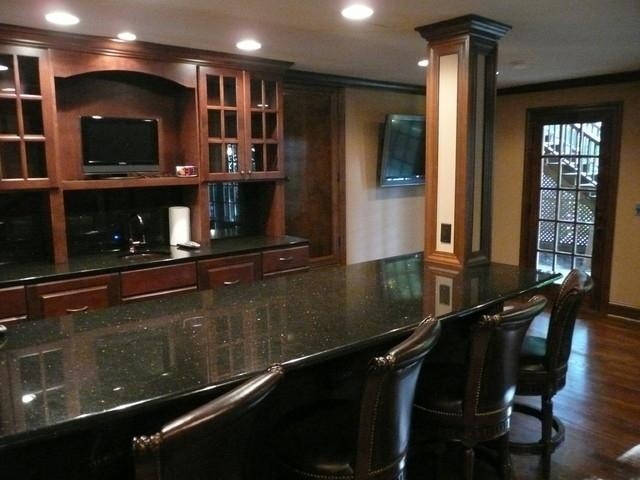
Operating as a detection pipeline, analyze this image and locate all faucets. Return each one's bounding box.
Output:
[128,213,143,248]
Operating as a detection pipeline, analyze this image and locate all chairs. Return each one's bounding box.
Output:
[295,313,442,479]
[126,364,318,479]
[414,269,596,479]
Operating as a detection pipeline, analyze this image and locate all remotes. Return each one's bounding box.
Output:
[176,243,193,248]
[186,240,201,248]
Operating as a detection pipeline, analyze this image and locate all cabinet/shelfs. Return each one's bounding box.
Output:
[0,24,314,325]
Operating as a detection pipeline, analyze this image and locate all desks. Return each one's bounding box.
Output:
[3,262,563,446]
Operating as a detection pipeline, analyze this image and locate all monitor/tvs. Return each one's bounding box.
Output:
[81,115,160,179]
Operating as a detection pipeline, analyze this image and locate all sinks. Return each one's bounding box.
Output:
[119,252,169,262]
